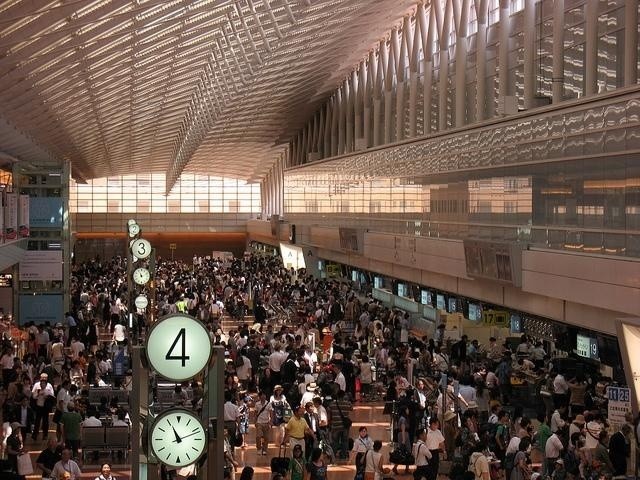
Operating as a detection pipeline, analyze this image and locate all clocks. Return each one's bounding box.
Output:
[149,407,209,468]
[132,267,151,285]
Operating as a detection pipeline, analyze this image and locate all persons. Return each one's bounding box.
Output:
[1,251,403,480]
[402,312,639,480]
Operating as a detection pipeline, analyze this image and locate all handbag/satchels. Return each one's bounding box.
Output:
[343,418,352,429]
[235,429,243,446]
[389,445,415,465]
[17,453,33,475]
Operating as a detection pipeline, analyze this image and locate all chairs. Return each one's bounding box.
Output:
[79,380,204,464]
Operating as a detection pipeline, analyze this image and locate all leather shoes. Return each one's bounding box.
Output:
[393,467,411,475]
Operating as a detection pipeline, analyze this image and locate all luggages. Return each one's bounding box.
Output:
[271,443,290,473]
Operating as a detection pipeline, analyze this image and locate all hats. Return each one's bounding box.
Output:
[11,422,22,431]
[307,382,319,391]
[573,415,585,424]
[468,401,478,408]
[64,312,70,316]
[444,411,456,421]
[555,460,563,464]
[273,385,284,391]
